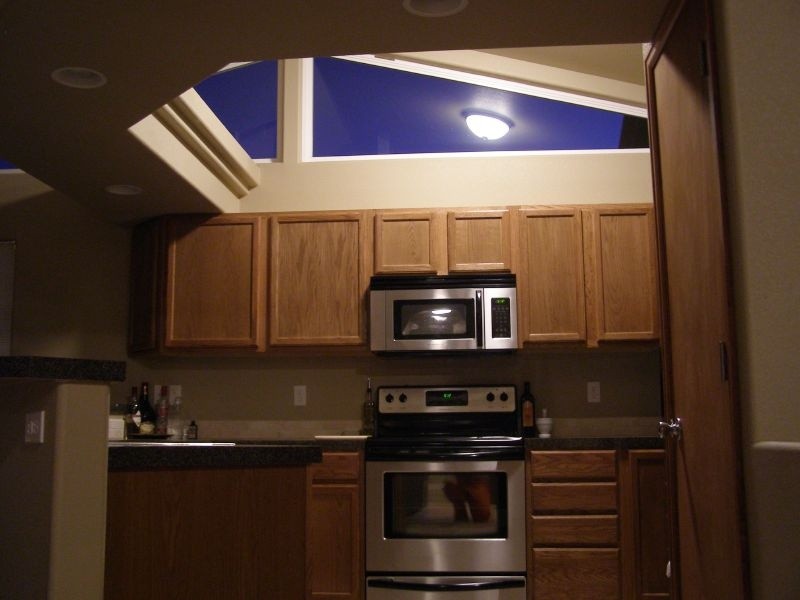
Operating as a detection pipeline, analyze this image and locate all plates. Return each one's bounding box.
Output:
[314,434,368,440]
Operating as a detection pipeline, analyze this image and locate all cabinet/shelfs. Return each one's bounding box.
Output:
[145,204,682,600]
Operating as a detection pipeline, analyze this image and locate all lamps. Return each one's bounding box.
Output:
[463,108,514,141]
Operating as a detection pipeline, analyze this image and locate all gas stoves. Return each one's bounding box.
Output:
[367,385,523,446]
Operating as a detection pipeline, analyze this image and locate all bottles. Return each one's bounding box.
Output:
[140,384,155,433]
[169,397,186,441]
[156,385,168,434]
[363,377,375,436]
[187,420,198,440]
[129,433,174,440]
[127,384,141,434]
[520,380,537,438]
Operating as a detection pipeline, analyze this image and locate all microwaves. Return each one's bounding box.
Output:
[370,273,519,353]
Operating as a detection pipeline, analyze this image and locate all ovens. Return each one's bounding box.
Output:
[363,448,530,600]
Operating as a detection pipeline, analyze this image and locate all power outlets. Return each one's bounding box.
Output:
[25,412,44,445]
[587,381,600,402]
[295,386,306,406]
